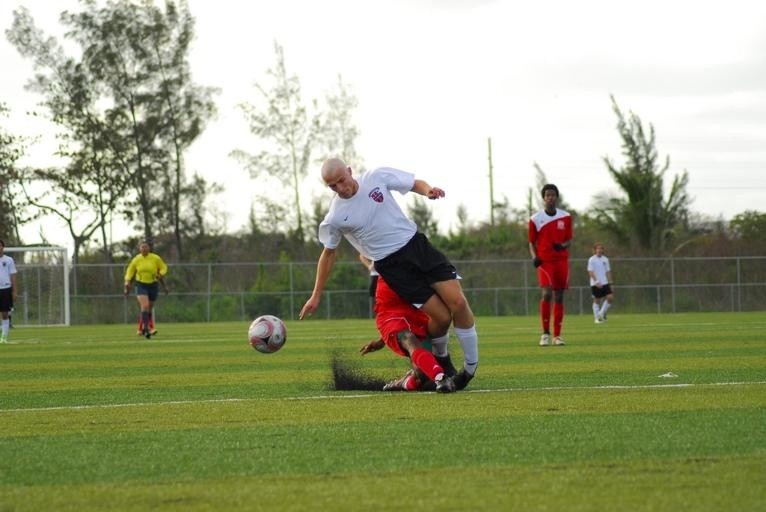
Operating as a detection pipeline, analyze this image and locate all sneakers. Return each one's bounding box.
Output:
[382,353,475,393]
[137,328,158,338]
[552,336,565,346]
[594,310,608,323]
[539,334,550,346]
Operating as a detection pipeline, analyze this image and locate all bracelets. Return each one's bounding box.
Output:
[595,281,600,284]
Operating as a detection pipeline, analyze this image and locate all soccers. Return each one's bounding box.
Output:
[248,316,286,353]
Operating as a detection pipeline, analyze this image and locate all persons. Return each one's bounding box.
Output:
[299,158,479,391]
[358,255,456,394]
[527,184,573,346]
[123,269,169,336]
[586,243,616,323]
[8,304,16,328]
[0,240,18,344]
[125,240,168,339]
